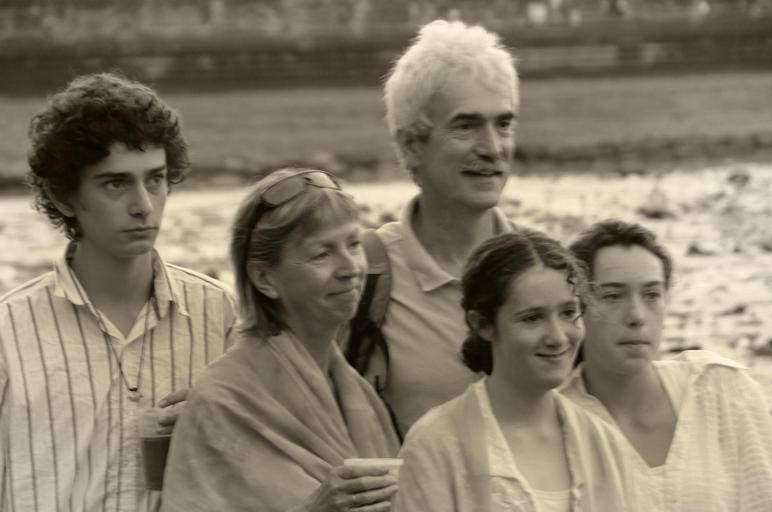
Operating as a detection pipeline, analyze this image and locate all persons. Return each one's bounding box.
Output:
[390,228,672,512]
[338,19,536,438]
[161,166,406,512]
[0,71,245,512]
[560,218,772,512]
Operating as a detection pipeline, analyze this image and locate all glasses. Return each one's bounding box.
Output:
[242,169,344,278]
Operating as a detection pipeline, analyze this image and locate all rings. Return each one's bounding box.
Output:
[352,494,358,505]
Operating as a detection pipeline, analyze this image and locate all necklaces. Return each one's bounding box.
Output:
[90,293,151,403]
